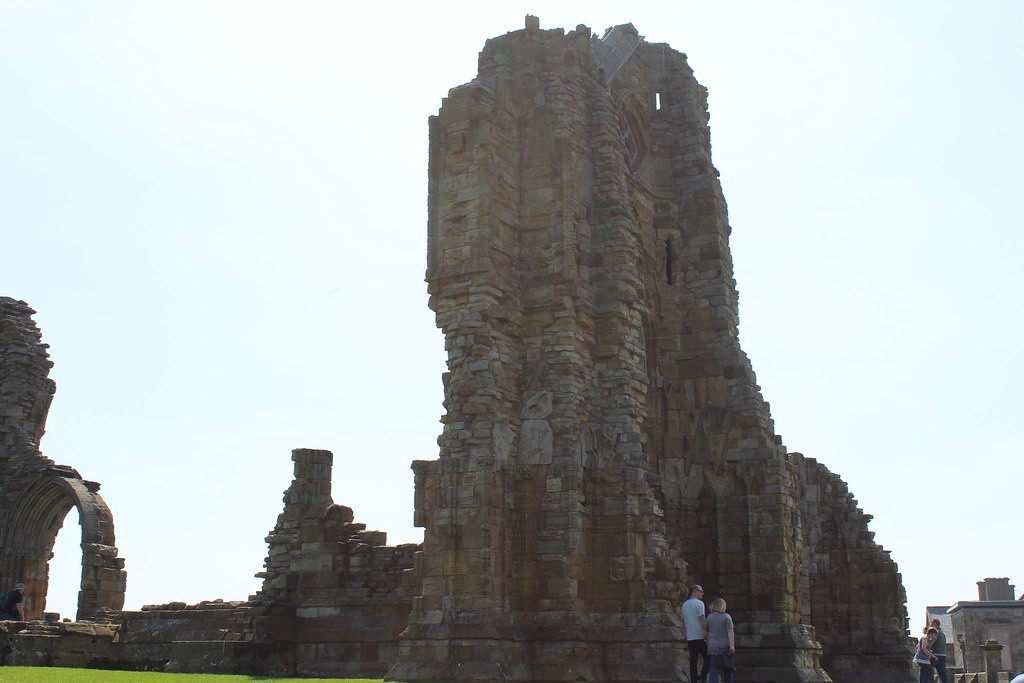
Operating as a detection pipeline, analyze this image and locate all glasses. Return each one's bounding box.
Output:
[694,589,704,593]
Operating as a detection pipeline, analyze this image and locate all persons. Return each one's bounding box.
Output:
[913,627,939,682]
[922,626,928,636]
[929,618,947,683]
[703,597,737,683]
[3,582,28,621]
[680,583,711,683]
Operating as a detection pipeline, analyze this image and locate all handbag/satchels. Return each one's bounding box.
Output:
[717,650,737,672]
[930,657,942,666]
